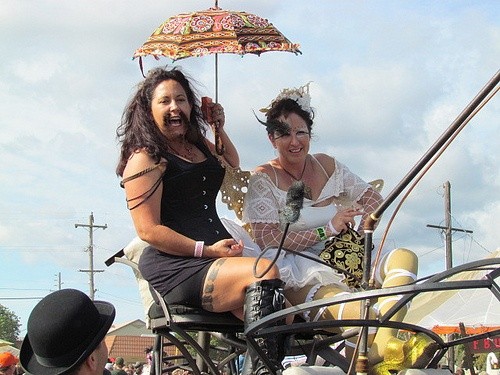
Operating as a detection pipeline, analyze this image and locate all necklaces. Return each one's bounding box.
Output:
[166,141,197,161]
[275,155,310,182]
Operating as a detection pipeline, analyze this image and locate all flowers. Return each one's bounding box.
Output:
[270,80,312,112]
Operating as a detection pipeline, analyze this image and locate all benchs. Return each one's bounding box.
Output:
[113,236,338,375]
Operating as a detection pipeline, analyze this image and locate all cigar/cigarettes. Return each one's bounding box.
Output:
[353,206,357,212]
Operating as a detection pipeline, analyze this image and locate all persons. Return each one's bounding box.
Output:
[113,63,295,375]
[0,348,242,375]
[245,80,441,375]
[20,288,116,375]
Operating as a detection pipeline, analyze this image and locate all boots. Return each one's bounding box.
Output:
[241,279,285,375]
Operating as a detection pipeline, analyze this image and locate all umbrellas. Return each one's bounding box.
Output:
[132,0,303,156]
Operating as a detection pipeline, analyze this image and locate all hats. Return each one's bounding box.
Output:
[19,289,116,374]
[0,351,18,367]
[135,361,143,367]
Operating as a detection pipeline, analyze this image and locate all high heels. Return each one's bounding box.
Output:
[369,331,437,375]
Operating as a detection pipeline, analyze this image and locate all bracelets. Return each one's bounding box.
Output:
[316,226,327,241]
[193,240,204,257]
